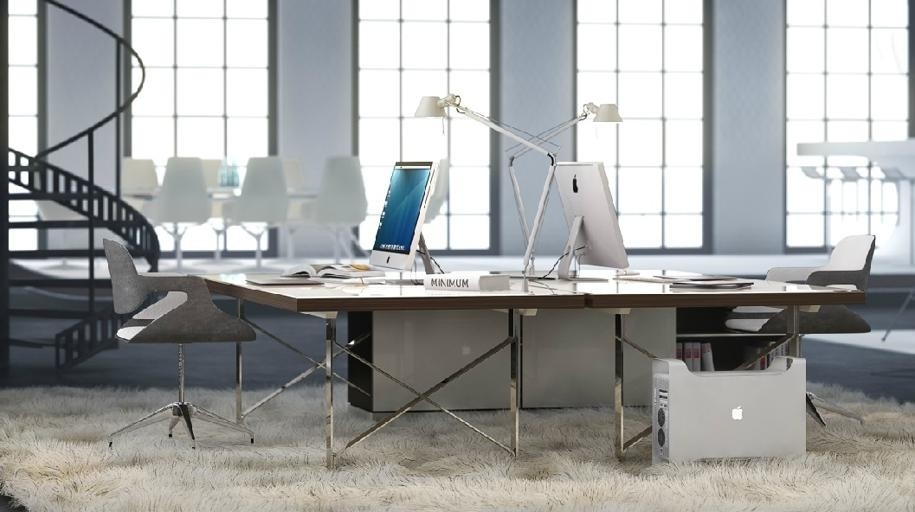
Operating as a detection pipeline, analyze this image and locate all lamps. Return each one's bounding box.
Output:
[415,89,625,283]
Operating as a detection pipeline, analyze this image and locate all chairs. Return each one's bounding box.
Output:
[106,241,261,448]
[112,153,374,264]
[769,238,875,422]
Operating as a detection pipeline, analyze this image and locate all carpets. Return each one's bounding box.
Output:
[1,384,914,512]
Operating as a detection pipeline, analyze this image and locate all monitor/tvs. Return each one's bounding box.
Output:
[368,162,444,279]
[553,161,629,282]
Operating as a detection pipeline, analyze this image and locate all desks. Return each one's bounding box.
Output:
[510,275,868,462]
[191,268,585,471]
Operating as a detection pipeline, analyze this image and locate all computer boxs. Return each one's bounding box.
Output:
[651,355,806,467]
